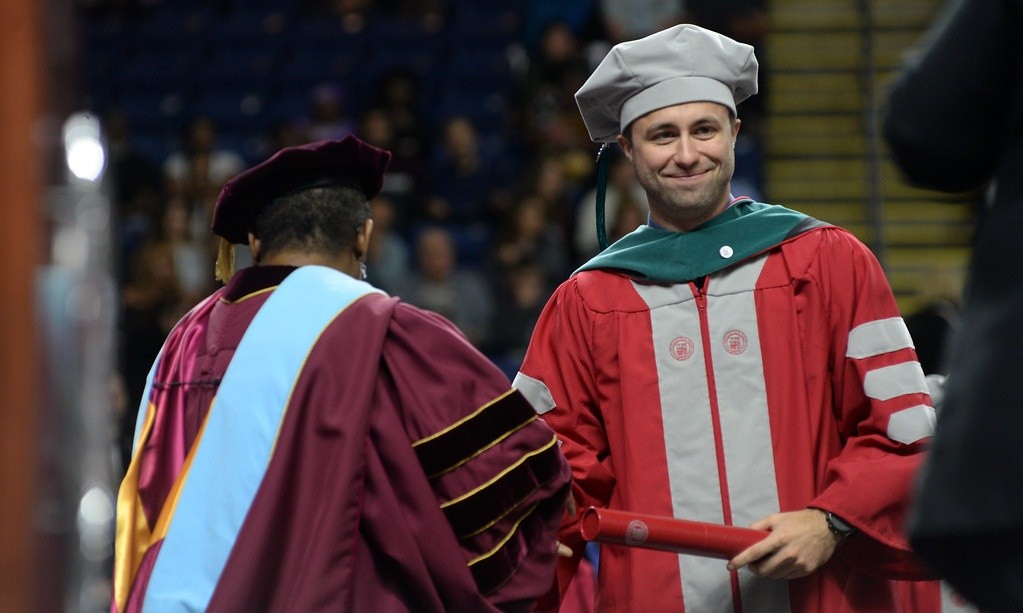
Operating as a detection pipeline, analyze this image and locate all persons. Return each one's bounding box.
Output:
[878,0,1023,613]
[127,115,651,350]
[512,22,934,613]
[110,136,573,613]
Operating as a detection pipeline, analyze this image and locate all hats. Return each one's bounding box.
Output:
[574,24,759,253]
[210,135,391,284]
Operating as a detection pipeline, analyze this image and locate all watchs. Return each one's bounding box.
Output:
[826,511,859,538]
